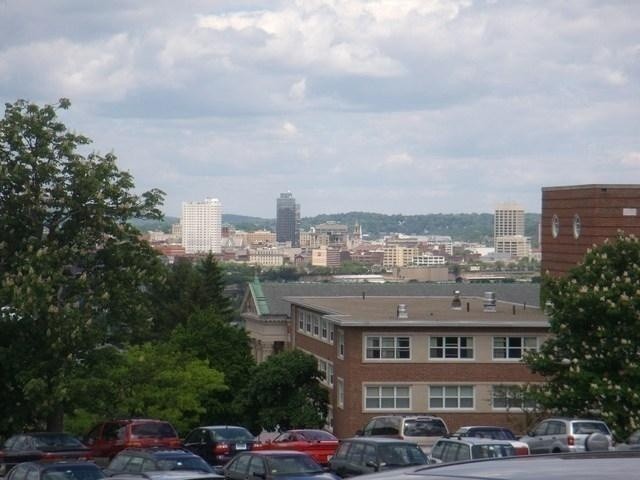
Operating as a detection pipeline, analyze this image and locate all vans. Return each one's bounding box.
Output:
[81,418,182,461]
[354,414,450,456]
[327,438,436,479]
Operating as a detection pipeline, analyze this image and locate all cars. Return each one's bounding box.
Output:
[308,448,640,480]
[180,426,262,467]
[0,432,96,477]
[611,426,640,452]
[430,425,530,456]
[260,429,340,464]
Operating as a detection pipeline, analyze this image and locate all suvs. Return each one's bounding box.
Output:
[425,434,516,466]
[517,417,612,455]
